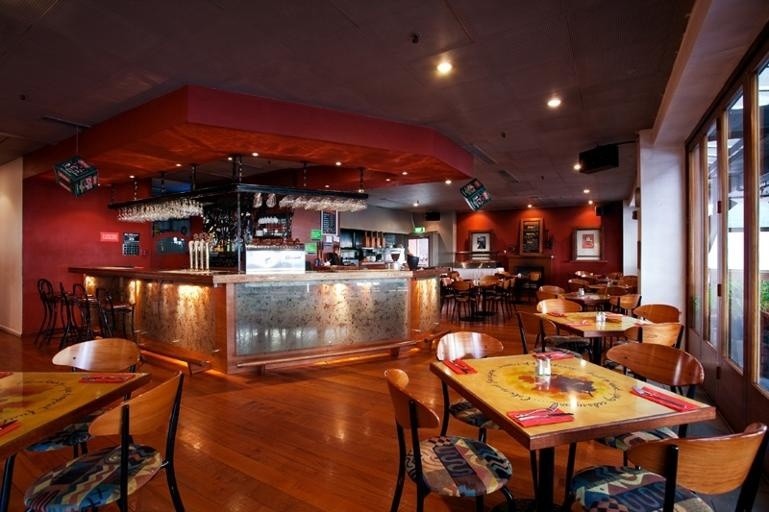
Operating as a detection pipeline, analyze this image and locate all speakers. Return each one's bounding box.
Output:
[595,206,615,216]
[580,144,619,175]
[424,213,441,220]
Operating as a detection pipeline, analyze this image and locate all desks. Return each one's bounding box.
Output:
[533,311,656,365]
[0,370,152,463]
[429,351,717,512]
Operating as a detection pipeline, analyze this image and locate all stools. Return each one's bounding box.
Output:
[32,278,136,350]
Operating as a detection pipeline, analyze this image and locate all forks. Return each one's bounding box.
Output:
[633,385,686,409]
[517,402,559,419]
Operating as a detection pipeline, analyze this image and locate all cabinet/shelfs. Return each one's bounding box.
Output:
[68,265,450,375]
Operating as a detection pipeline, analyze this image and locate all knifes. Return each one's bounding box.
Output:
[519,413,574,420]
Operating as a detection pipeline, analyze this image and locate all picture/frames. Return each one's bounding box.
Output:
[572,227,606,261]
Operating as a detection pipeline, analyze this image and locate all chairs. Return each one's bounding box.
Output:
[632,304,682,323]
[481,275,499,313]
[607,287,626,296]
[537,285,566,301]
[0,336,142,512]
[496,277,517,316]
[516,310,582,358]
[610,271,623,280]
[452,281,478,321]
[534,298,593,362]
[435,330,505,443]
[564,343,705,499]
[608,294,642,319]
[620,275,638,293]
[567,278,590,292]
[623,322,685,349]
[561,421,769,512]
[441,277,461,318]
[23,369,185,512]
[574,270,590,280]
[383,368,514,512]
[487,279,510,319]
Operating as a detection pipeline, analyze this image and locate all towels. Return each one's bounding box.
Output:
[78,372,135,384]
[546,311,567,318]
[563,319,592,327]
[630,385,700,412]
[442,358,477,375]
[531,349,575,362]
[0,371,12,378]
[0,418,23,437]
[506,406,574,428]
